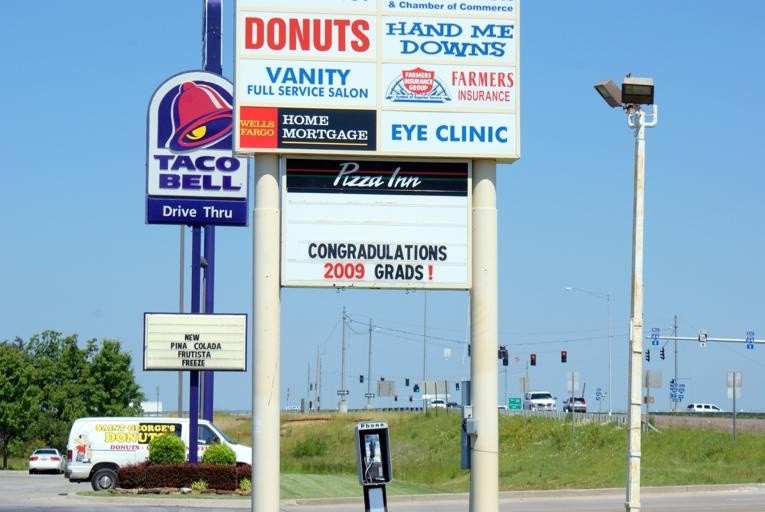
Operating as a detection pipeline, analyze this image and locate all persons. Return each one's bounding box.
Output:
[74,434,87,463]
[86,444,93,463]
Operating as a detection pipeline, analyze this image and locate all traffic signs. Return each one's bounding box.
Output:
[668,382,687,402]
[509,397,522,409]
[595,384,602,402]
[744,331,757,349]
[651,327,661,344]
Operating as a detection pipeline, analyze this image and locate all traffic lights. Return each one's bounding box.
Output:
[561,351,567,363]
[530,353,536,366]
[414,387,419,393]
[645,348,650,362]
[659,347,664,360]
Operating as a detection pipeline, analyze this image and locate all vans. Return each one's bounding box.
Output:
[687,404,722,412]
[63,417,252,494]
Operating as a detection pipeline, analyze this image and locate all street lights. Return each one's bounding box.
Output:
[595,68,657,512]
[366,316,382,412]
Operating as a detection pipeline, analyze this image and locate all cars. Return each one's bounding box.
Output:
[432,399,458,410]
[28,449,65,475]
[499,405,509,413]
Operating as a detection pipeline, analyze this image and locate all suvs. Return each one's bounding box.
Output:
[563,397,587,414]
[523,391,557,412]
